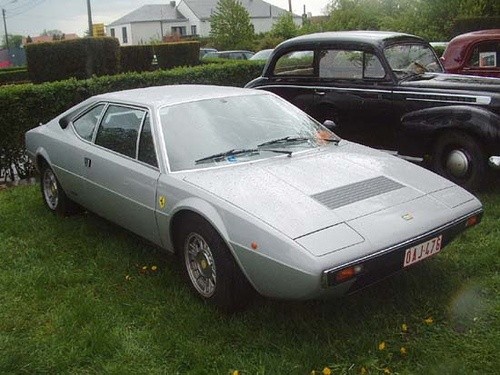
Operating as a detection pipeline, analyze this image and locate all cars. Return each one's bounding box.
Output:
[25,85,483,303]
[199,48,217,64]
[250,48,276,63]
[204,51,255,65]
[428,33,500,80]
[243,30,500,192]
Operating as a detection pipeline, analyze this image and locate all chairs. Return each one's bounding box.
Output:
[165,104,224,172]
[104,112,149,162]
